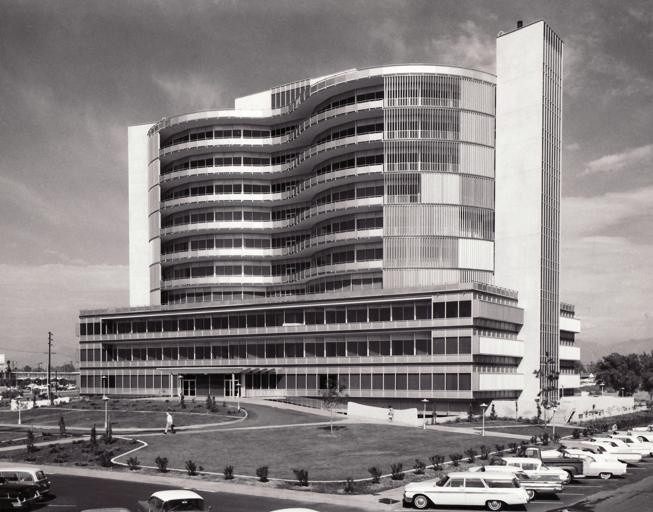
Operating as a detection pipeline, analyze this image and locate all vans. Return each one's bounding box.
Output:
[402,472,529,511]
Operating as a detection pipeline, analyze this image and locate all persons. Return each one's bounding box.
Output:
[163,411,174,434]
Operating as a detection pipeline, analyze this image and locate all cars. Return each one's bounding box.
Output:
[0,462,51,512]
[462,422,652,502]
[134,488,212,512]
[56,383,78,391]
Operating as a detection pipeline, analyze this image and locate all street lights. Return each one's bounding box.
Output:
[480,402,488,435]
[177,374,184,399]
[420,398,429,428]
[101,396,108,427]
[552,403,558,439]
[235,382,241,411]
[101,375,106,398]
[15,394,22,423]
